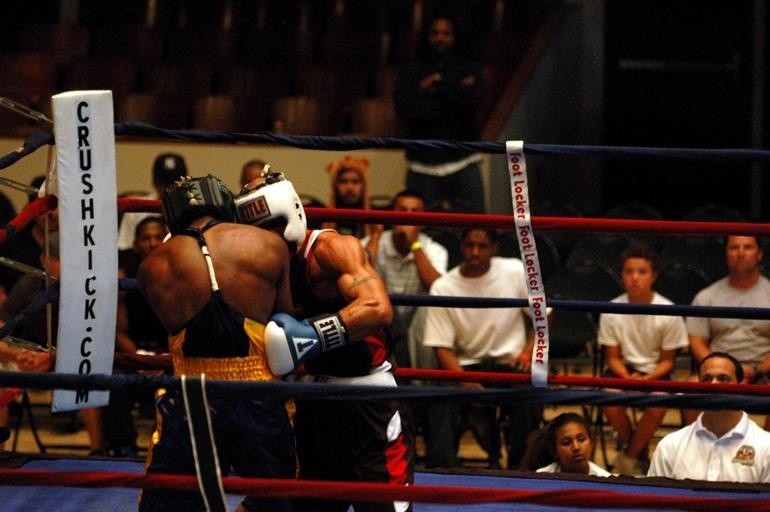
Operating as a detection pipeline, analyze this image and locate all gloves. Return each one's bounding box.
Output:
[263,312,349,376]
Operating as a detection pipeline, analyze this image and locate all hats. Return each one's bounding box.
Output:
[153,154,187,184]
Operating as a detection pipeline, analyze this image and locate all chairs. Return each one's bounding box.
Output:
[1,254,770,470]
[0,0,533,154]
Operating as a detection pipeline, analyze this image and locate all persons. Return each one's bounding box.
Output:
[646,352,769,484]
[426,222,554,471]
[682,233,770,431]
[598,242,689,478]
[393,13,487,213]
[1,150,449,512]
[515,413,618,476]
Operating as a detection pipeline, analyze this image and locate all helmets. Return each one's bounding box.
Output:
[162,174,233,230]
[235,179,307,255]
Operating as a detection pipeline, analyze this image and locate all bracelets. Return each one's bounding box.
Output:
[756,368,763,379]
